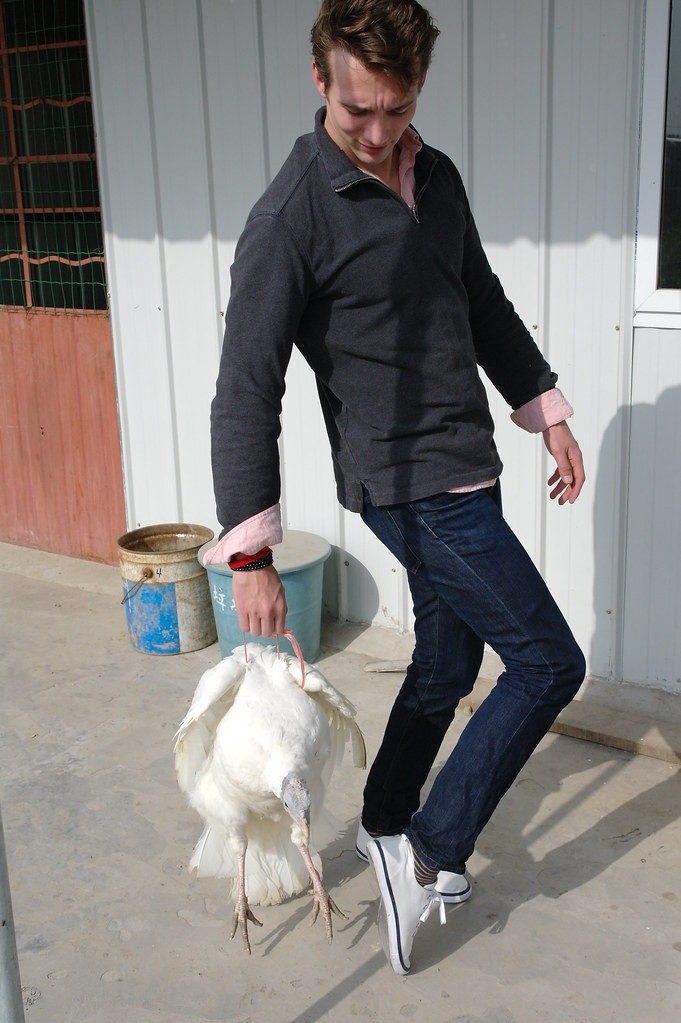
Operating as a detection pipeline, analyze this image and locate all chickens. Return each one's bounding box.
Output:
[172,643,368,956]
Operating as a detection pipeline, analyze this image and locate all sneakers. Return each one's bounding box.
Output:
[354,820,471,904]
[366,834,439,976]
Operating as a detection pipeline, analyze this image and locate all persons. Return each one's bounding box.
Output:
[202,0,587,976]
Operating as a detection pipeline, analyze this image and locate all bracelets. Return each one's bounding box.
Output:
[228,549,276,572]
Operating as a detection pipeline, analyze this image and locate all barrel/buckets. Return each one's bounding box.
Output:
[197,531,331,665]
[117,522,214,656]
